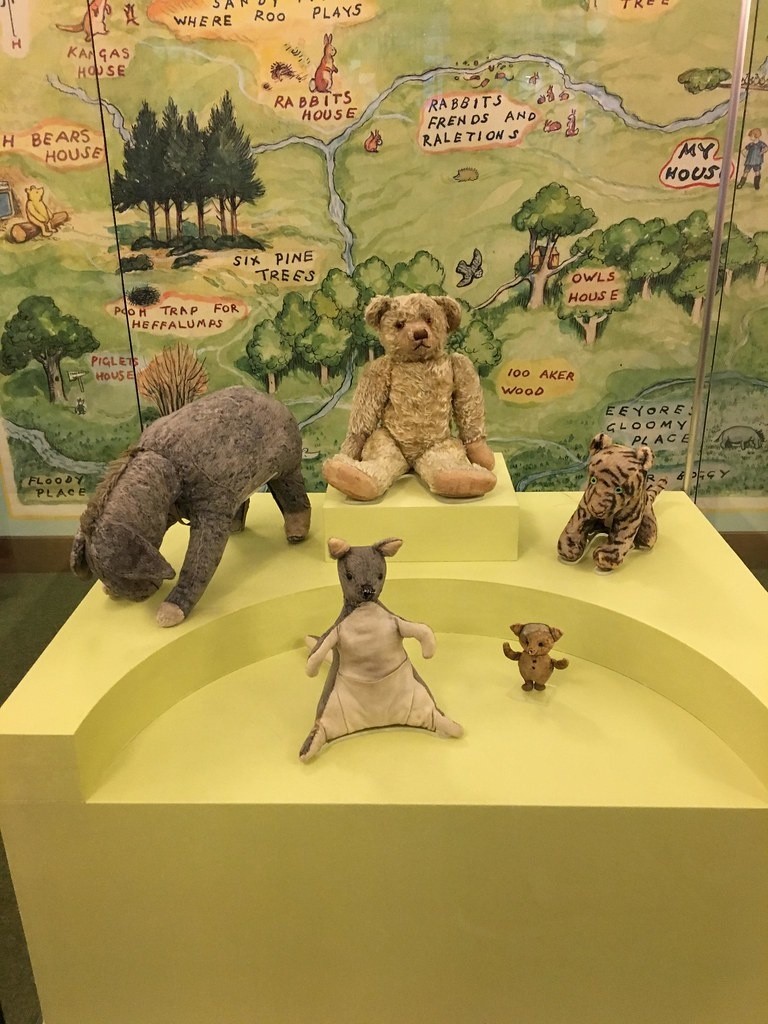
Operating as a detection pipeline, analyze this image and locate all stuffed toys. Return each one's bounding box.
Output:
[70,384,312,627]
[554,436,666,570]
[322,293,498,502]
[300,538,465,762]
[503,623,568,691]
